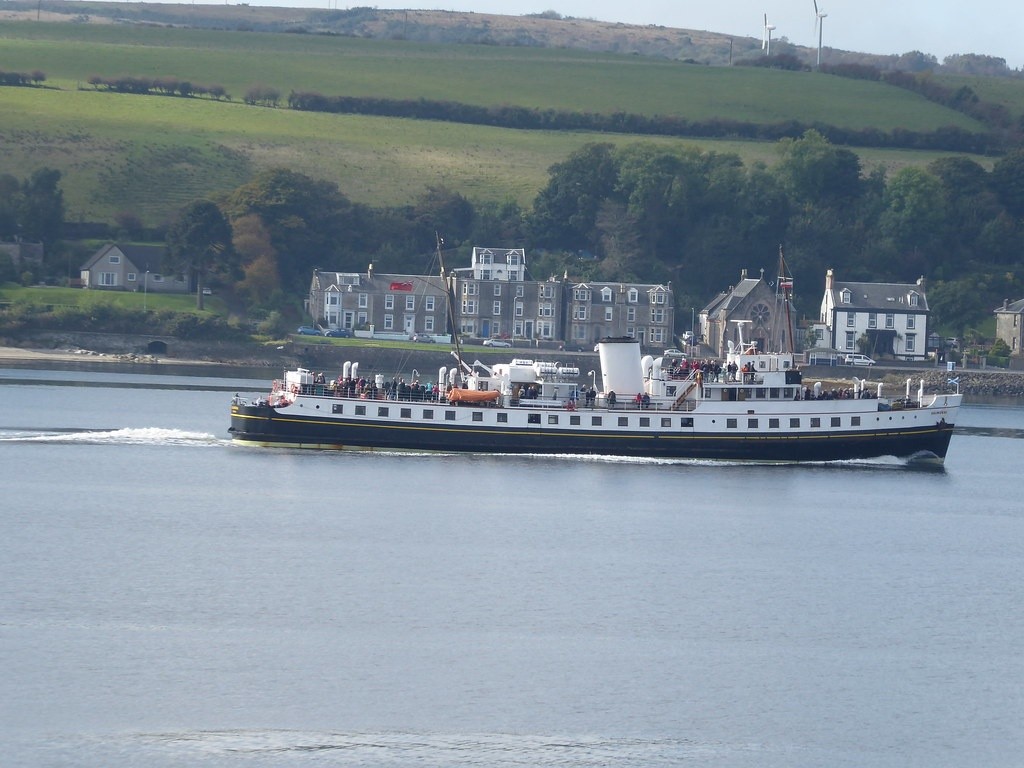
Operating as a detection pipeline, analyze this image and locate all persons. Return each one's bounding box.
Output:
[518,386,526,407]
[722,359,738,382]
[741,360,758,384]
[312,370,325,396]
[571,386,580,408]
[526,386,533,407]
[581,383,590,408]
[257,396,263,406]
[607,390,616,409]
[382,376,439,403]
[494,389,503,408]
[331,374,378,399]
[462,380,468,403]
[642,391,650,409]
[891,395,919,409]
[268,379,297,406]
[670,356,690,380]
[636,392,643,410]
[589,386,596,408]
[691,357,721,383]
[447,382,459,406]
[531,385,539,407]
[795,386,878,400]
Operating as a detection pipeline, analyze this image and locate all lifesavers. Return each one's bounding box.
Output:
[291,384,295,392]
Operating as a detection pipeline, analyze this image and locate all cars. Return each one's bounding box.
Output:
[325,328,351,338]
[944,337,959,348]
[203,287,211,296]
[482,339,511,348]
[559,344,585,352]
[297,326,321,336]
[664,349,687,358]
[414,333,436,343]
[683,331,695,340]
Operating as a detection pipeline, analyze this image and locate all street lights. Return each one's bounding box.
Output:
[691,308,695,357]
[144,271,150,311]
[512,297,518,347]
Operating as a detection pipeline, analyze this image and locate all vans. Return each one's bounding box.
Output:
[845,354,876,366]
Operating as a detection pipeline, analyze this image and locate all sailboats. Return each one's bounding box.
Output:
[227,231,962,466]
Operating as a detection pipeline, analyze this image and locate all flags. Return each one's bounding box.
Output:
[389,282,412,292]
[796,365,801,376]
[779,277,793,288]
